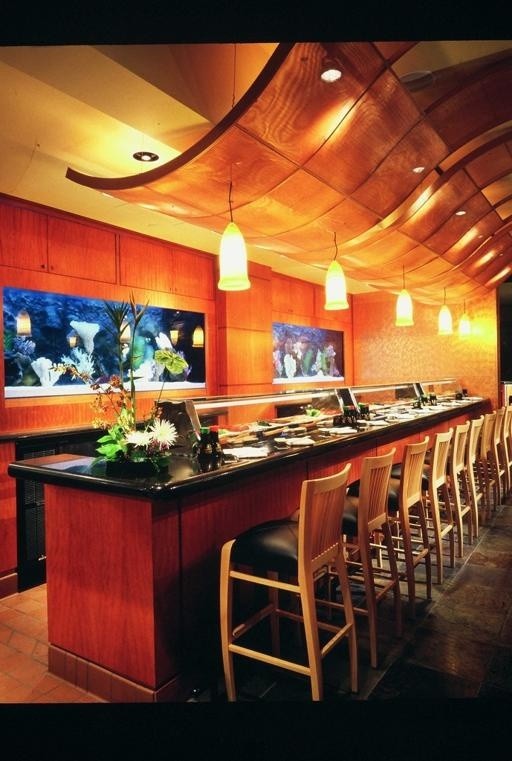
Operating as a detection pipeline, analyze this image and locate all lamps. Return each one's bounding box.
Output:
[215,180,251,293]
[15,305,205,351]
[322,232,347,312]
[436,285,453,336]
[393,264,415,327]
[458,299,470,335]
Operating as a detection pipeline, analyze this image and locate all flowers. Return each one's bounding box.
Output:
[47,292,188,467]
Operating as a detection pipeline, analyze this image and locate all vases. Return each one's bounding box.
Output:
[106,460,172,482]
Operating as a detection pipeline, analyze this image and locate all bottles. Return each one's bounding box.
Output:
[420,393,437,406]
[341,403,370,428]
[209,426,222,470]
[197,428,214,474]
